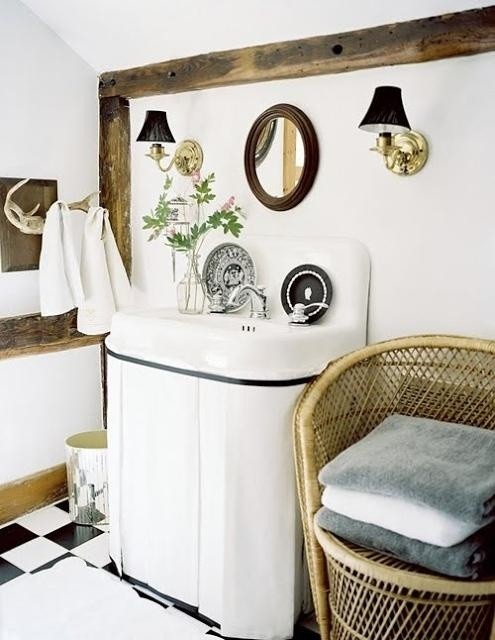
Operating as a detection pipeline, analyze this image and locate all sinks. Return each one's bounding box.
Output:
[144,312,286,335]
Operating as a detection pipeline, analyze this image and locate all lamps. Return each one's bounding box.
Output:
[358,85,428,176]
[136,110,203,176]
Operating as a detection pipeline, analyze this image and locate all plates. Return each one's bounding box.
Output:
[281,264,333,324]
[200,243,256,312]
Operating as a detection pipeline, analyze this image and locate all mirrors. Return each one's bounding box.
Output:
[243,103,319,211]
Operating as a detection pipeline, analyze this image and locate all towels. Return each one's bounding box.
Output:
[318,415,495,580]
[39,201,130,336]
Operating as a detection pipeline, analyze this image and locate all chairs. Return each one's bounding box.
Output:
[292,334,494,640]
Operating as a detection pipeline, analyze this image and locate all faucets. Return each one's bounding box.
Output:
[226,284,271,315]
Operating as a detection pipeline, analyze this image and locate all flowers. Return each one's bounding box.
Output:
[142,171,247,251]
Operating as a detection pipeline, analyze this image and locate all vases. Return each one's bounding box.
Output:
[177,254,206,314]
